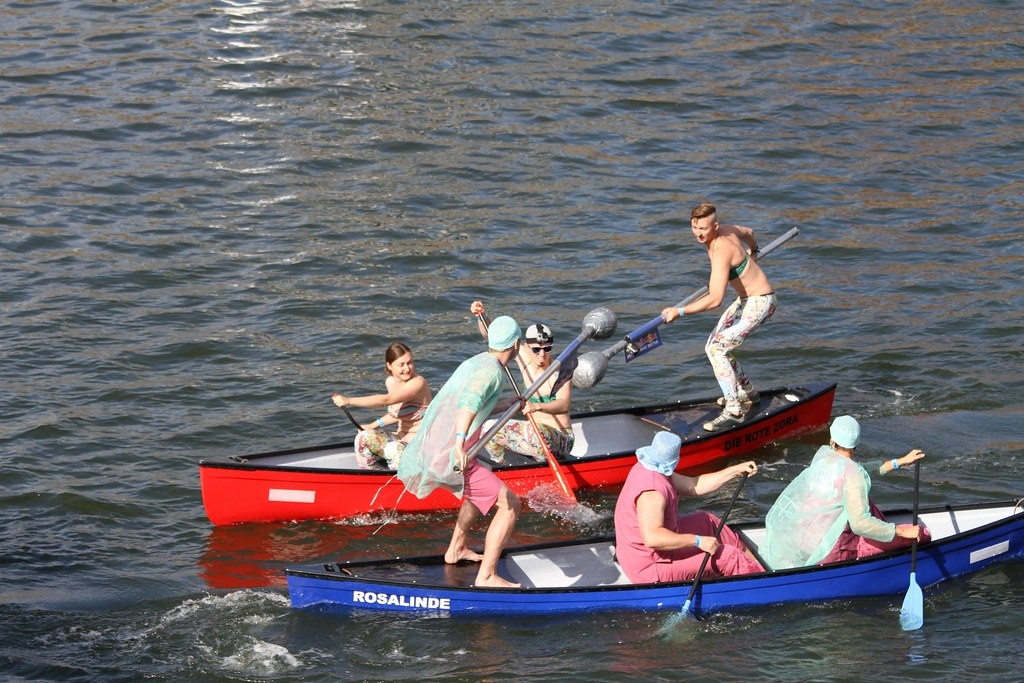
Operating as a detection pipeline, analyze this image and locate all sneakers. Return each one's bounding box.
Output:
[717,387,760,405]
[703,413,745,432]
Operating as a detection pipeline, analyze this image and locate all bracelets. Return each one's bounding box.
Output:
[696,534,701,548]
[376,416,385,429]
[892,458,898,470]
[679,307,684,318]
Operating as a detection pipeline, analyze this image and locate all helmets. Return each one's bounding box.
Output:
[525,323,553,343]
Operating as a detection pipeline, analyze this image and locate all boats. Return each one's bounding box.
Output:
[293,500,1024,615]
[198,381,838,526]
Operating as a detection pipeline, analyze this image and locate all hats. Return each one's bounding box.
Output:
[635,431,682,476]
[830,415,862,449]
[488,315,522,350]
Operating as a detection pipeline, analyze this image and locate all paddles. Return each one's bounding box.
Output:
[681,463,755,620]
[475,302,580,504]
[333,393,365,432]
[899,450,926,632]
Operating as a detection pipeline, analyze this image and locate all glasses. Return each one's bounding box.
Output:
[528,345,553,353]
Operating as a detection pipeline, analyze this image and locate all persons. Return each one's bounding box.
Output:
[416,315,527,590]
[797,416,931,565]
[471,300,574,465]
[614,430,766,585]
[660,203,777,430]
[332,342,432,470]
